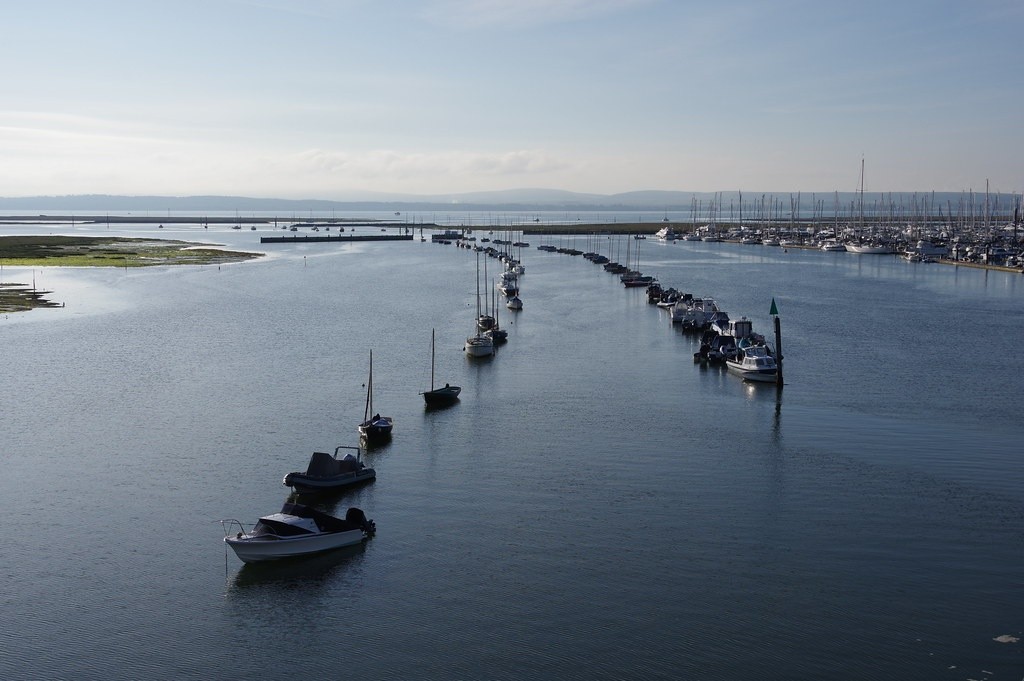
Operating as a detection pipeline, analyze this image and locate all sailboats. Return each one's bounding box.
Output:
[537,157,1024,384]
[280,213,531,357]
[419,327,462,405]
[358,349,395,444]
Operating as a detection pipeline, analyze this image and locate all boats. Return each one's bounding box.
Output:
[220,500,377,566]
[282,445,377,498]
